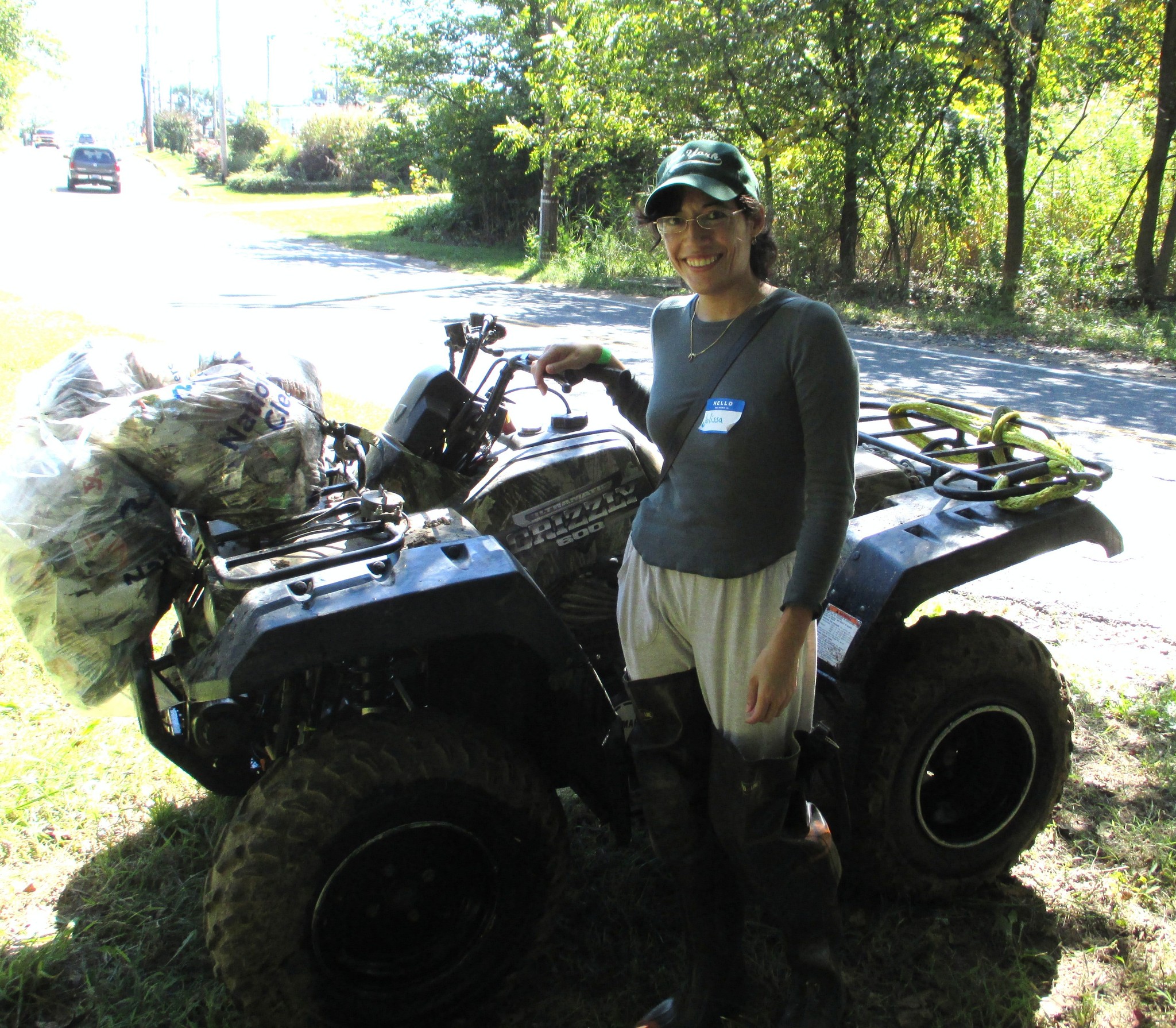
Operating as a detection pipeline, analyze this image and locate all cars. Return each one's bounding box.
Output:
[63,147,121,193]
[78,132,97,144]
[33,127,60,149]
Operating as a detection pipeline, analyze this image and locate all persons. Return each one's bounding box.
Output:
[528,141,861,1028]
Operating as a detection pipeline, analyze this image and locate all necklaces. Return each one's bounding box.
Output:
[688,287,756,363]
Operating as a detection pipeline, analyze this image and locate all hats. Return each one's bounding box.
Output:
[644,140,758,217]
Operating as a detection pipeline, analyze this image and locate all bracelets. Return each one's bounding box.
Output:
[596,347,611,366]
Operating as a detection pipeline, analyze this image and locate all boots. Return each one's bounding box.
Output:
[707,721,852,1028]
[621,665,748,1028]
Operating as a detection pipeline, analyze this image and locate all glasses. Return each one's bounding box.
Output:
[653,205,747,234]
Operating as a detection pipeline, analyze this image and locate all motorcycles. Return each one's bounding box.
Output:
[125,309,1116,1028]
